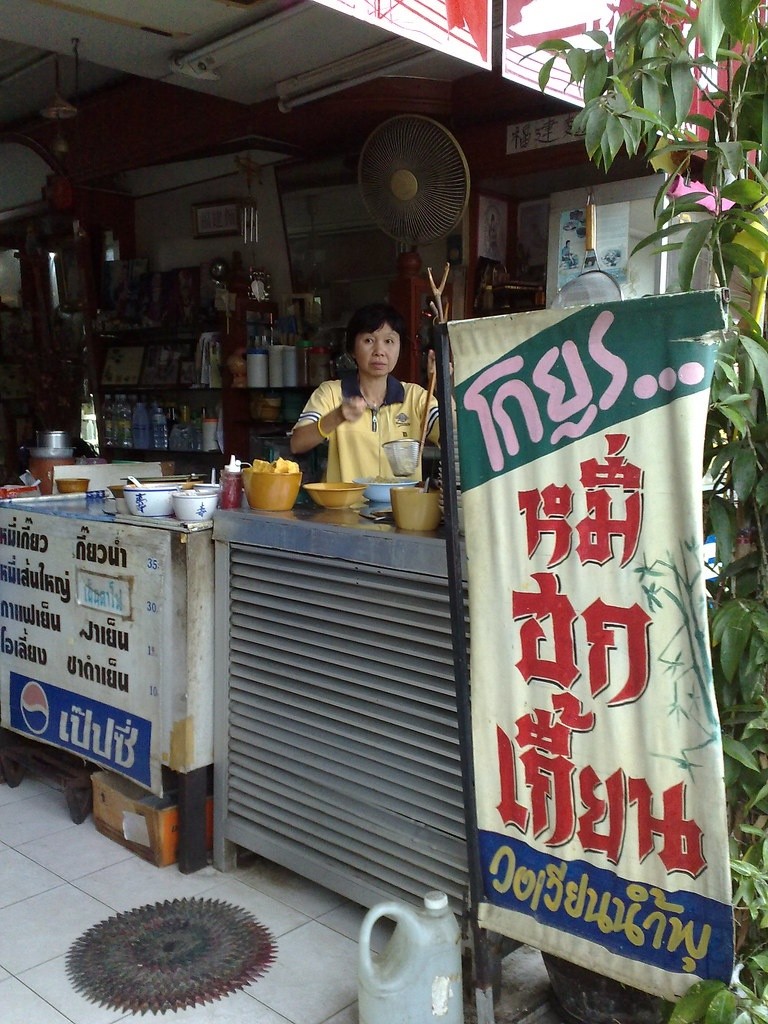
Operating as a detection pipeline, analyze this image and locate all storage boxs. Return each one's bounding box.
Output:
[88,769,213,869]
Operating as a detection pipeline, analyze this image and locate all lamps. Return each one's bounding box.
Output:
[277,35,442,112]
[36,60,78,155]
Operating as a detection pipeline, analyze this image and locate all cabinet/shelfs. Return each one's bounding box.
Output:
[91,294,340,480]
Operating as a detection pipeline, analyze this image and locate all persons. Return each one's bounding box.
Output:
[289,303,456,505]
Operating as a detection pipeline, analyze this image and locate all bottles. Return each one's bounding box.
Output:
[245,336,332,387]
[100,393,220,453]
[483,285,494,310]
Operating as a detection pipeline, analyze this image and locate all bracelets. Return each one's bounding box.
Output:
[318,415,334,438]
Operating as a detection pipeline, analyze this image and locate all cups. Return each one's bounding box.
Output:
[217,470,243,511]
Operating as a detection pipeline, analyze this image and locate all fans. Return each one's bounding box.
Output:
[356,114,470,248]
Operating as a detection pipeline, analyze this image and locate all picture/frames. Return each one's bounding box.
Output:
[470,187,518,277]
[191,198,244,238]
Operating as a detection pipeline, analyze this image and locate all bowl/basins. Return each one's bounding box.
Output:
[352,476,419,503]
[193,483,220,492]
[172,490,218,522]
[107,485,124,498]
[241,467,303,512]
[302,482,368,510]
[390,486,443,531]
[122,483,181,518]
[55,478,90,493]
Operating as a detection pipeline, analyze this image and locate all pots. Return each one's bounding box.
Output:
[36,430,71,447]
[20,446,77,458]
